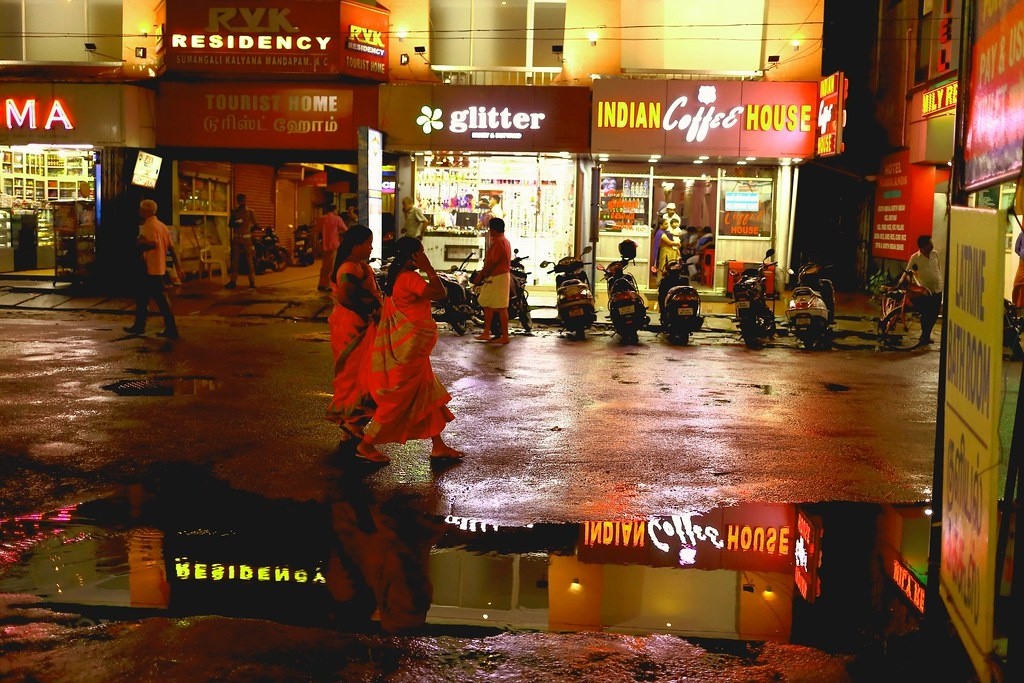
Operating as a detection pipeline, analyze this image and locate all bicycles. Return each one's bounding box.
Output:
[877,264,927,351]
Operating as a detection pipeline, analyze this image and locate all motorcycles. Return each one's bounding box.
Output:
[488,248,533,338]
[433,251,486,335]
[367,256,395,292]
[1001,297,1024,361]
[288,223,314,265]
[252,224,290,275]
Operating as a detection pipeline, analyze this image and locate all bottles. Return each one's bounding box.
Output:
[622,198,645,213]
[623,180,648,196]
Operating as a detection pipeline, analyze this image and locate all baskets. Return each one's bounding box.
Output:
[909,285,930,306]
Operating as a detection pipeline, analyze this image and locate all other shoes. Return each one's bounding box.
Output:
[250,281,256,288]
[338,434,357,445]
[123,325,145,334]
[225,281,236,288]
[339,421,365,442]
[156,328,178,338]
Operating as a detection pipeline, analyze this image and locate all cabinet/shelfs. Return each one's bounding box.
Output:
[601,177,649,230]
[0,148,96,288]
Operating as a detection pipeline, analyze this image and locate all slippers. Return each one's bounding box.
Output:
[430,450,464,460]
[354,452,392,463]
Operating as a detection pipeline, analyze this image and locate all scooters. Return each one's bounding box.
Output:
[650,252,704,347]
[785,259,837,351]
[540,246,597,342]
[727,247,777,351]
[596,247,650,345]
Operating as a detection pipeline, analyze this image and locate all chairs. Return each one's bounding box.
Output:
[701,249,714,288]
[199,245,231,284]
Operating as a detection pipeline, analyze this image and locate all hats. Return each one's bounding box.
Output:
[667,202,676,209]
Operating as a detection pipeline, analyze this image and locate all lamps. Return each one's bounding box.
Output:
[765,56,780,71]
[84,43,127,63]
[413,46,431,65]
[552,45,565,63]
[134,47,147,59]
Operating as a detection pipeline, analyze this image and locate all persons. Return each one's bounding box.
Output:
[660,202,681,228]
[223,194,261,289]
[474,217,511,344]
[654,219,681,289]
[122,198,184,338]
[399,196,429,241]
[898,236,942,351]
[666,218,683,261]
[687,226,713,281]
[679,225,699,262]
[461,193,505,230]
[339,203,359,226]
[353,237,466,464]
[328,226,384,448]
[316,203,349,291]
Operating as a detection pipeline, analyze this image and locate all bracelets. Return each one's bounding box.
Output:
[484,266,491,274]
